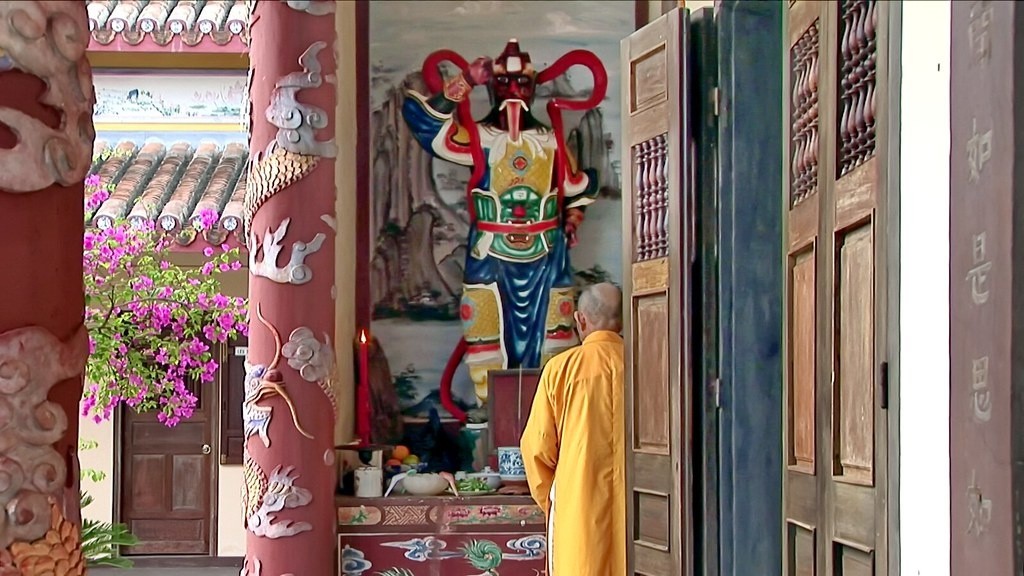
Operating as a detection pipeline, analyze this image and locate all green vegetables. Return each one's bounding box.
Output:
[446,478,491,491]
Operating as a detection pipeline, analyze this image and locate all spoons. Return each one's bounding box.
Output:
[384,473,407,498]
[440,471,460,497]
[401,469,416,494]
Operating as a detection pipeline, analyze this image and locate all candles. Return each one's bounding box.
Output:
[355,328,372,440]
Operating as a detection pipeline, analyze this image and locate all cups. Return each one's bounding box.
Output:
[353,466,383,498]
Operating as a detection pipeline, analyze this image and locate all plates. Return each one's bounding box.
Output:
[444,488,496,496]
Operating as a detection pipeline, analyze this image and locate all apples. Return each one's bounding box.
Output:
[387,445,419,465]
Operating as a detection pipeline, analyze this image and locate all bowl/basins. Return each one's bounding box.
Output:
[383,462,428,472]
[467,474,502,489]
[402,474,449,496]
[497,446,526,481]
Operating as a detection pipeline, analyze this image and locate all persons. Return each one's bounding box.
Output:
[519,282,629,576]
[405,36,604,425]
